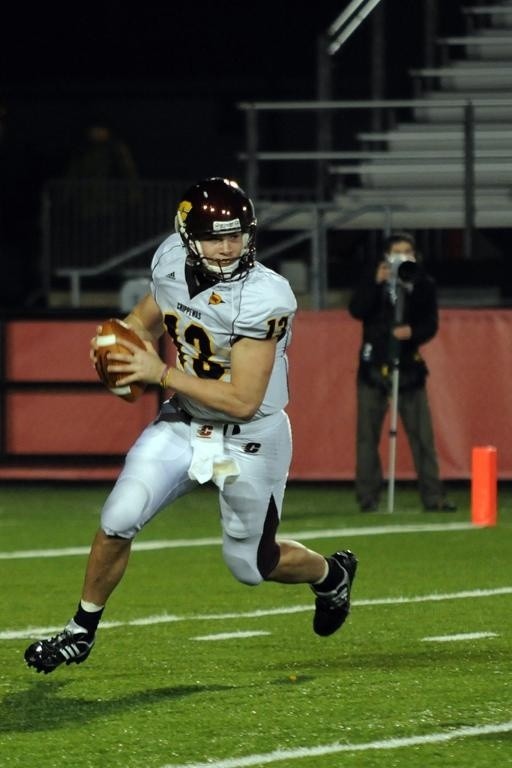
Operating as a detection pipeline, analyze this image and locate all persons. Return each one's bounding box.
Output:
[24,173,359,675]
[346,230,459,516]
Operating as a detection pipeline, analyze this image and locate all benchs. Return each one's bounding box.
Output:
[340,1,511,203]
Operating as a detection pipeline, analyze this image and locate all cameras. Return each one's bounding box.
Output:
[386,253,420,289]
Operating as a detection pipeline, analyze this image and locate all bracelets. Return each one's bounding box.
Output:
[160,365,178,388]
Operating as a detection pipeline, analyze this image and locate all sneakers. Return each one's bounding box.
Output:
[310,548,359,637]
[23,617,96,675]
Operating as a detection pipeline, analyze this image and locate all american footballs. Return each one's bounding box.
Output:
[94,320,149,402]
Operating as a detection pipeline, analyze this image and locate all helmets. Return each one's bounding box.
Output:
[173,176,258,258]
